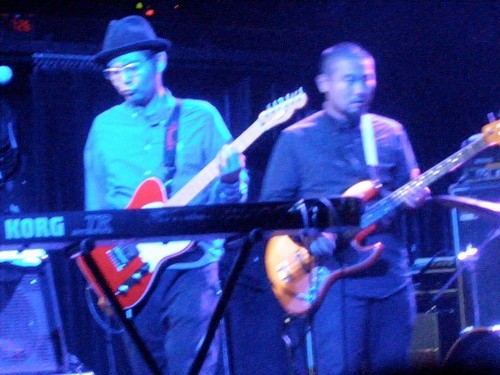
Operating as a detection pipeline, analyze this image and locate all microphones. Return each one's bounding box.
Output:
[0,66,14,85]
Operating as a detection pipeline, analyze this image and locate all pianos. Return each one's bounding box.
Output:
[0,196,364,375]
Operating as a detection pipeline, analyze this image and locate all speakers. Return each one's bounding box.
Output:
[407,311,457,375]
[450,183,500,330]
[0,253,69,375]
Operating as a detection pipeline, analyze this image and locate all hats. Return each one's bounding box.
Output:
[92,15,170,62]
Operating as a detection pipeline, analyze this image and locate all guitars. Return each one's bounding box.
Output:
[263,112,500,317]
[72,86,309,316]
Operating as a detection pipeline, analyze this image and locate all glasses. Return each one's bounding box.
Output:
[102,53,154,78]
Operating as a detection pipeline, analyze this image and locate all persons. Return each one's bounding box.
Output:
[259,41,431,375]
[82,16,250,375]
